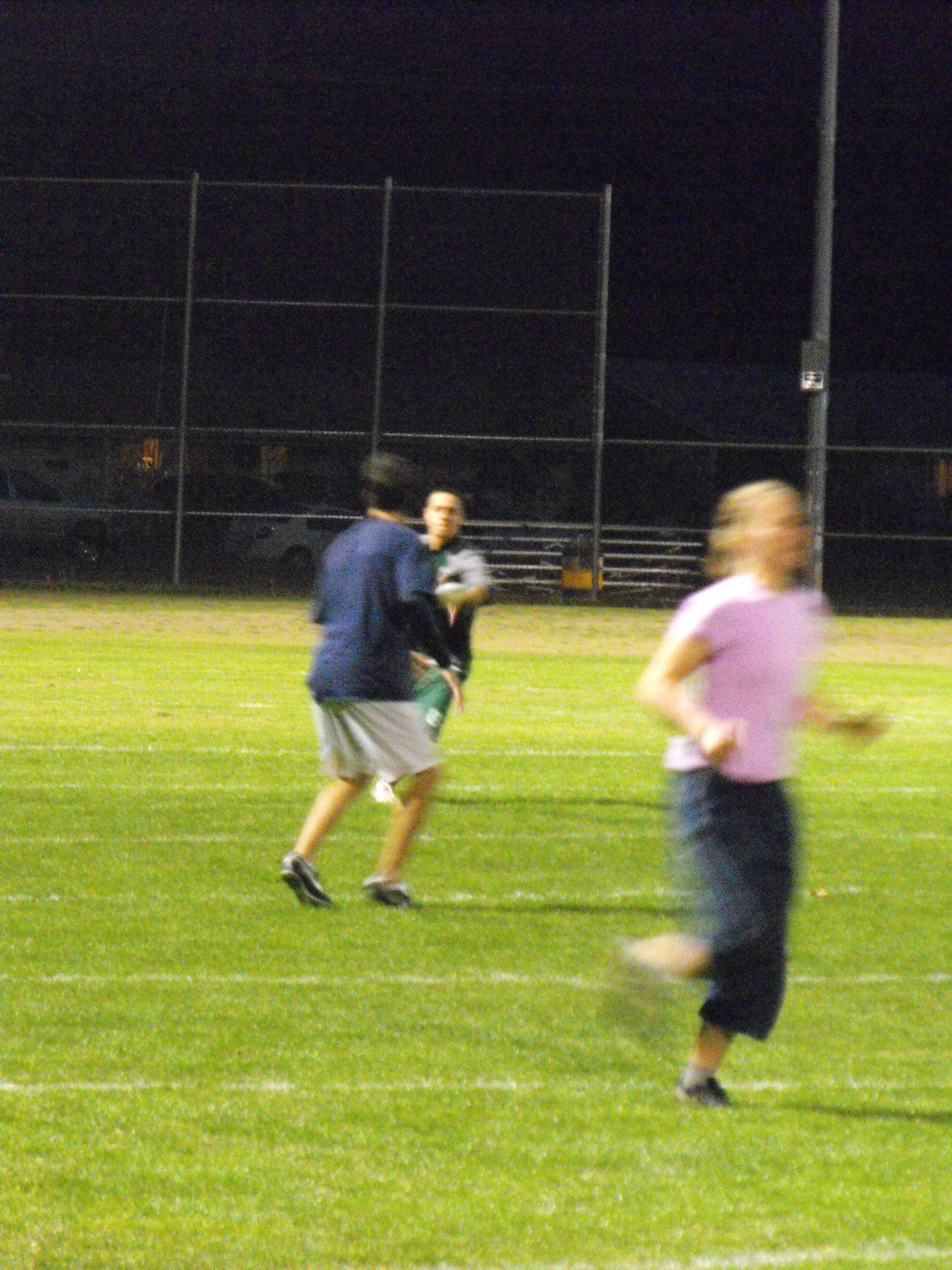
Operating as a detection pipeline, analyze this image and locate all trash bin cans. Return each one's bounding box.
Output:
[561,542,604,598]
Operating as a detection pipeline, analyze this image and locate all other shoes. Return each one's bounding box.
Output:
[370,780,397,804]
[673,1076,728,1109]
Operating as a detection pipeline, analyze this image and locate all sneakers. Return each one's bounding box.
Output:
[360,879,422,911]
[279,856,332,906]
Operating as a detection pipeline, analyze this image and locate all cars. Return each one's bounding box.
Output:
[227,499,358,593]
[0,470,107,568]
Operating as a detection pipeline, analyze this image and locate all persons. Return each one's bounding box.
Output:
[275,448,468,913]
[373,489,492,803]
[615,482,884,1111]
